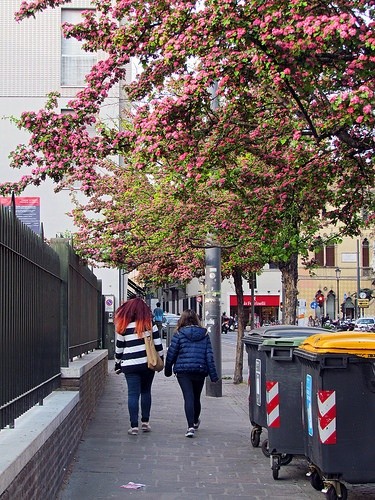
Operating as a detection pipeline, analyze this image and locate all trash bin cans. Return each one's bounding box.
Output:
[241,326,300,456]
[166,317,180,350]
[293,332,375,500]
[259,326,334,479]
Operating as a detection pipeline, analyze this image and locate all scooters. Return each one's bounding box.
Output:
[230,324,235,331]
[222,321,230,334]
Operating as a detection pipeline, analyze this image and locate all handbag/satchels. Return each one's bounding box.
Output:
[144,335,164,371]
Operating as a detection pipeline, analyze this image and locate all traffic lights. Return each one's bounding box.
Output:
[316,290,324,308]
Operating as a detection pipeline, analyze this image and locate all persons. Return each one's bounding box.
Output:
[164,309,219,435]
[114,297,164,435]
[153,302,163,338]
[308,313,329,327]
[221,311,238,332]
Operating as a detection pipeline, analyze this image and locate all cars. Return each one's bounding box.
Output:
[353,318,375,331]
[161,312,181,339]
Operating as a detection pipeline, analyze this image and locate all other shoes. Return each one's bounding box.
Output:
[194,418,202,430]
[185,428,195,437]
[127,427,138,435]
[141,422,151,432]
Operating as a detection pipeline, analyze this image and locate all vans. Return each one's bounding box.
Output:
[234,315,246,329]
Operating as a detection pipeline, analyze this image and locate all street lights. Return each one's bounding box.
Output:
[335,268,341,326]
